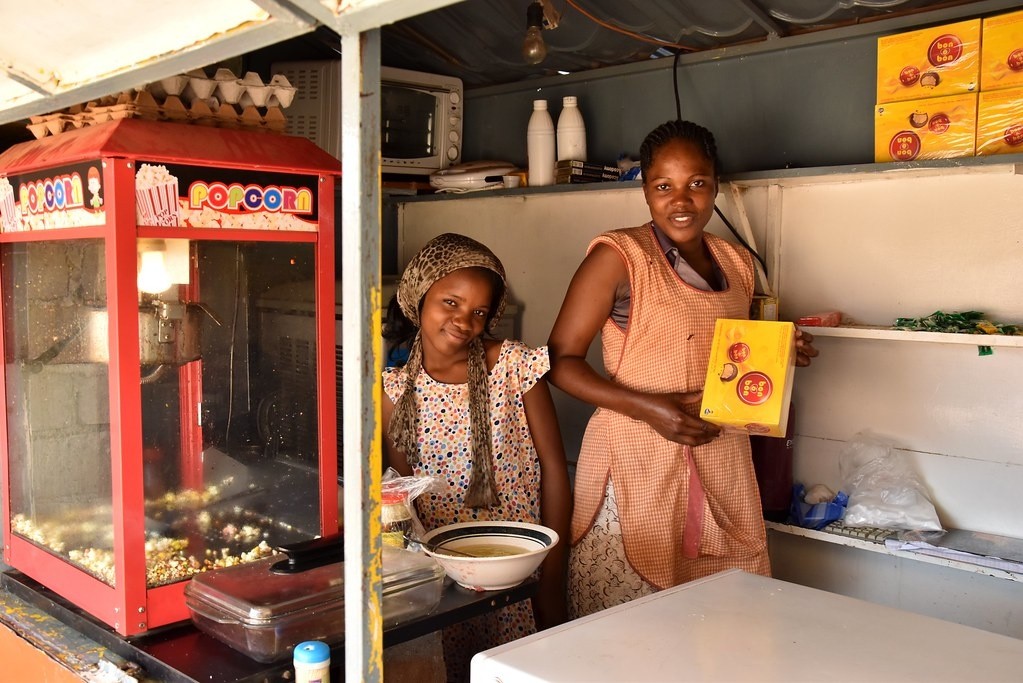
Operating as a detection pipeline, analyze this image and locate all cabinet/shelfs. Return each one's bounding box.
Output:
[394,152,1023,207]
[767,516,1023,581]
[770,315,1023,355]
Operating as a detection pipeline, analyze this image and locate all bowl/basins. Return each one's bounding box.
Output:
[420,521,561,591]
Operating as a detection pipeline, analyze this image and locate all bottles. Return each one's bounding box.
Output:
[557,96,586,162]
[527,99,555,185]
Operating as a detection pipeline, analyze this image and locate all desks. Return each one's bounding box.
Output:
[3,560,562,683]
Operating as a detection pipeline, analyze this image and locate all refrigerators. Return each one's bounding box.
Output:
[471,566,1023,683]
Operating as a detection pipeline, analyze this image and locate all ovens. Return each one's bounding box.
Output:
[271,61,464,176]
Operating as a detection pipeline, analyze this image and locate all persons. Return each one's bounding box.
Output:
[544,122,770,624]
[372,232,572,683]
